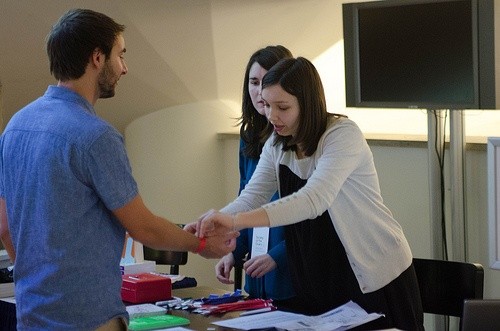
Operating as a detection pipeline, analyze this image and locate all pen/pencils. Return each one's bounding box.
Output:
[240,306,277,316]
[155,297,192,305]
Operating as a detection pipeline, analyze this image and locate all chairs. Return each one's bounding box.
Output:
[143,224,188,275]
[413,258,500,331]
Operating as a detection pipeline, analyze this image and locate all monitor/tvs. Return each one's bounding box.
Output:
[342,0,500,110]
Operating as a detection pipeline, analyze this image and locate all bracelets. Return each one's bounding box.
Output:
[231,215,236,231]
[191,238,206,254]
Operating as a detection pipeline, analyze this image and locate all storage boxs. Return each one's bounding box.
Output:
[122,273,172,303]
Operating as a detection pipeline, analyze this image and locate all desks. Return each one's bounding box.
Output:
[123,285,246,331]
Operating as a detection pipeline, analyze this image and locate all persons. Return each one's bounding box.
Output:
[183,56,424,331]
[215,46,294,299]
[0,8,240,331]
